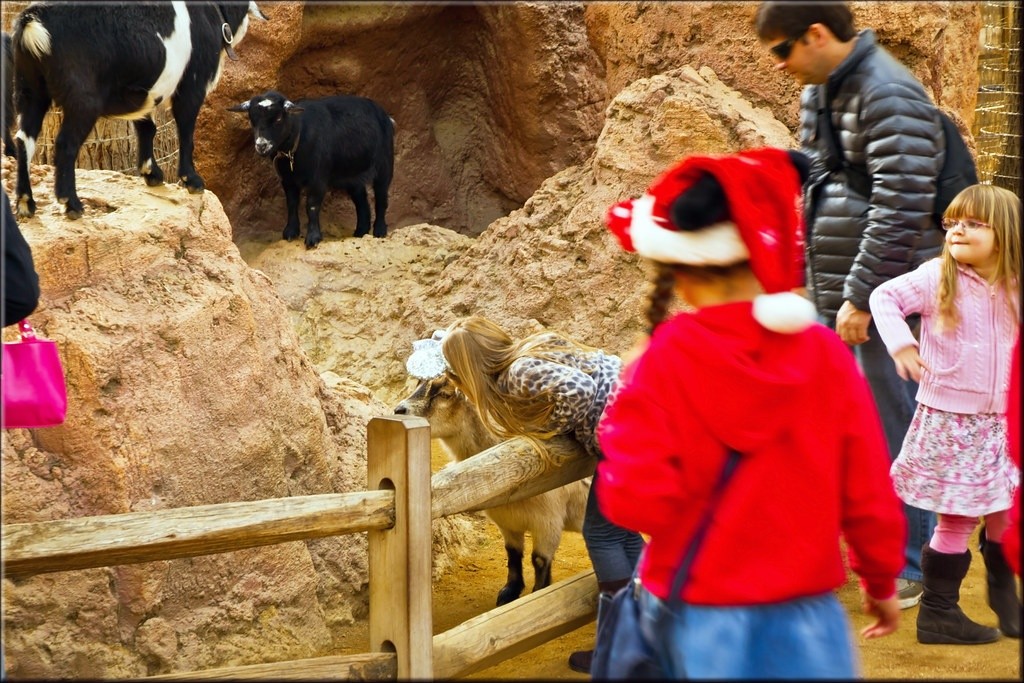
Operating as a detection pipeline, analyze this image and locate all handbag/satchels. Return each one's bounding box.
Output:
[589,576,678,680]
[818,80,979,235]
[0,319,67,428]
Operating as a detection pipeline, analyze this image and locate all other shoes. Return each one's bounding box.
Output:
[894,576,925,609]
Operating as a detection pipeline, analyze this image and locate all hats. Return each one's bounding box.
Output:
[611,146,817,335]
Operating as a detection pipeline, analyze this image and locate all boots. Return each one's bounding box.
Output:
[979,527,1022,638]
[915,545,1001,645]
[568,577,631,674]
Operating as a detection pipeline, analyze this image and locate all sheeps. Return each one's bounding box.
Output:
[228,91,400,257]
[10,0,272,220]
[392,327,593,607]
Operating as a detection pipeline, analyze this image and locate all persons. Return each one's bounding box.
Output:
[2,190,40,328]
[869,185,1023,644]
[598,149,906,679]
[441,315,644,673]
[752,1,946,609]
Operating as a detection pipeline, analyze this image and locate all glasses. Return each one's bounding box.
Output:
[770,20,816,61]
